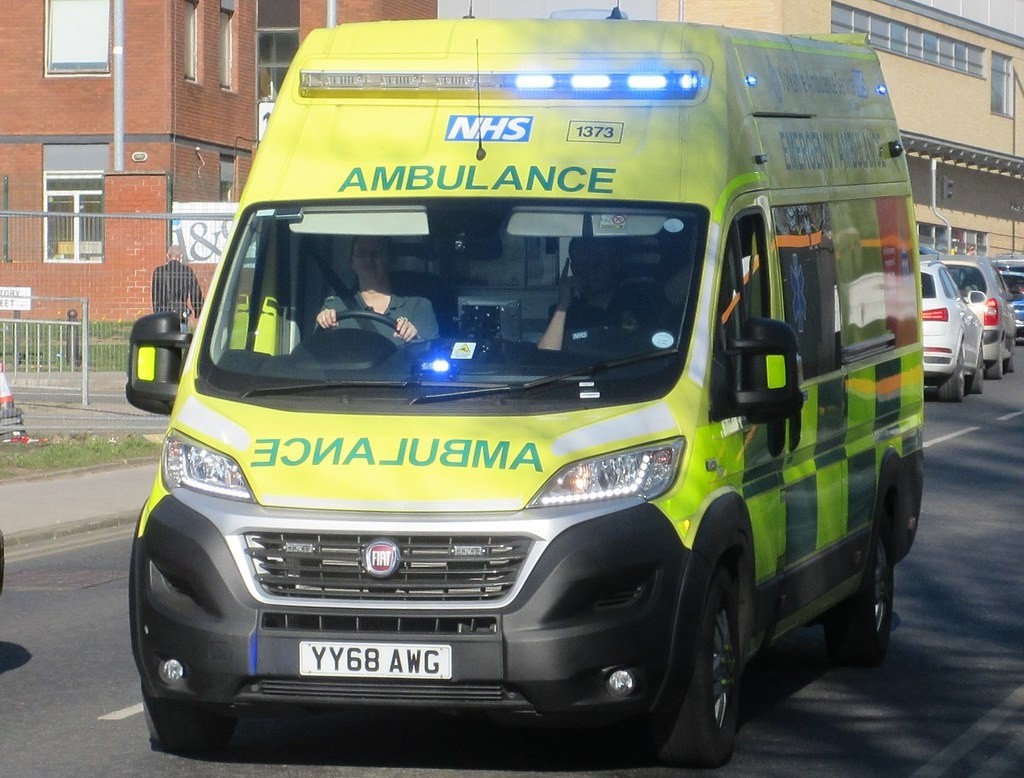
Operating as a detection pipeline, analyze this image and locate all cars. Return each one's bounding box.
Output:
[992,262,1024,346]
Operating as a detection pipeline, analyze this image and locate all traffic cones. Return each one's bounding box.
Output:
[0,361,29,444]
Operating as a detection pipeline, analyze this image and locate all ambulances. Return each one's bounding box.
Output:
[123,16,930,768]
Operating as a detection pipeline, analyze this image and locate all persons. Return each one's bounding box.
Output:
[966,245,975,256]
[536,238,650,352]
[151,245,204,333]
[316,236,440,346]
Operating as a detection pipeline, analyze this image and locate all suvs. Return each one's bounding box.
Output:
[920,261,985,402]
[935,256,1016,380]
